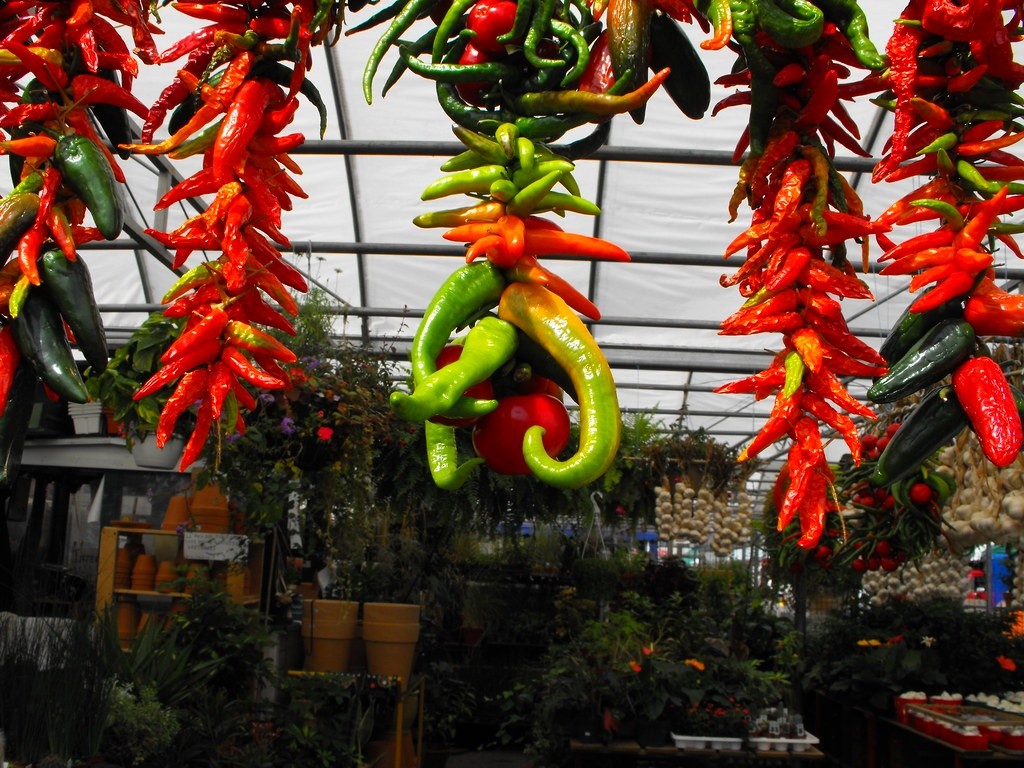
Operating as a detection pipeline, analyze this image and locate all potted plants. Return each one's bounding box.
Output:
[102,309,236,470]
[299,503,429,692]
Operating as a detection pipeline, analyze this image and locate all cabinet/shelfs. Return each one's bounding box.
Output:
[93,526,261,651]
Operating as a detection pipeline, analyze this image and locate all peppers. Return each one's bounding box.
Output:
[0,0,1024,582]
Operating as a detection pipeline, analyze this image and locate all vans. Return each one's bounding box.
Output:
[963,570,1011,617]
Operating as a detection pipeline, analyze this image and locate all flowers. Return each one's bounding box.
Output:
[209,293,416,521]
[672,697,754,738]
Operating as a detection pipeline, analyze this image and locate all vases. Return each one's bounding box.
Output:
[68,397,127,435]
[113,474,265,649]
[670,734,743,750]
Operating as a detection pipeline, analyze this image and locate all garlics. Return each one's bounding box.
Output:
[656,430,1024,611]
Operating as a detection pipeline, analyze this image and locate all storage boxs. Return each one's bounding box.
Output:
[895,691,1024,751]
